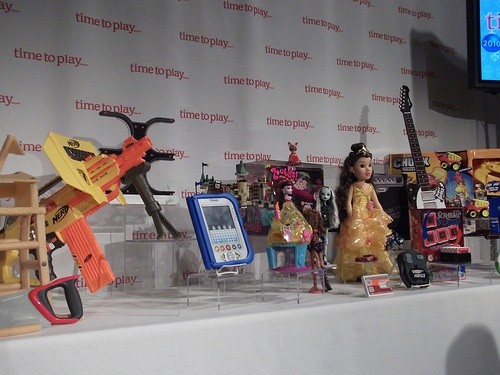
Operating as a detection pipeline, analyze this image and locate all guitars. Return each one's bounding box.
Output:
[398,83,448,209]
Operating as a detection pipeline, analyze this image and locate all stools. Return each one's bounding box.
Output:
[267,244,307,269]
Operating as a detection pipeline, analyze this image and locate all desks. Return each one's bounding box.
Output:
[187,273,257,311]
[427,262,460,287]
[261,266,325,304]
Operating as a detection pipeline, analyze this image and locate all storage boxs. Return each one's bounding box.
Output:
[440,252,471,263]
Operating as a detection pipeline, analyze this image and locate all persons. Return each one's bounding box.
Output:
[267,181,313,243]
[336,142,397,283]
[314,185,340,265]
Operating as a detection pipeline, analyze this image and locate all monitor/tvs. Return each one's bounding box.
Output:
[465,0,500,91]
